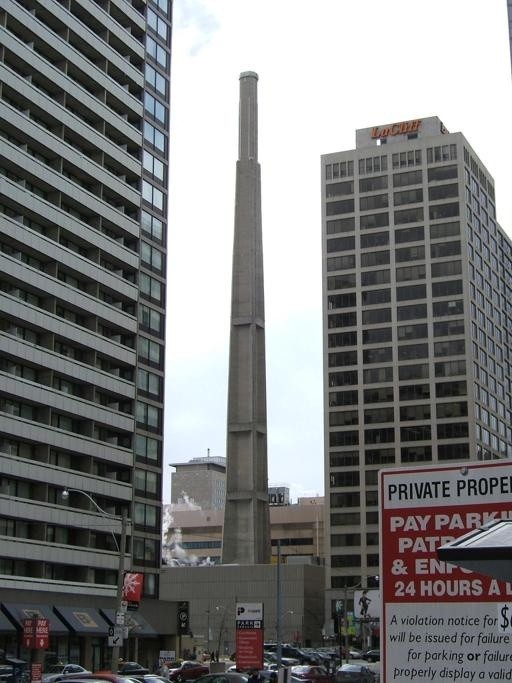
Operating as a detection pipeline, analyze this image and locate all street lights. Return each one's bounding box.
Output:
[61,487,128,675]
[343,575,379,663]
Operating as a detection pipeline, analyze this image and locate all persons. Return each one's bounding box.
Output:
[359,591,371,618]
[176,650,216,664]
